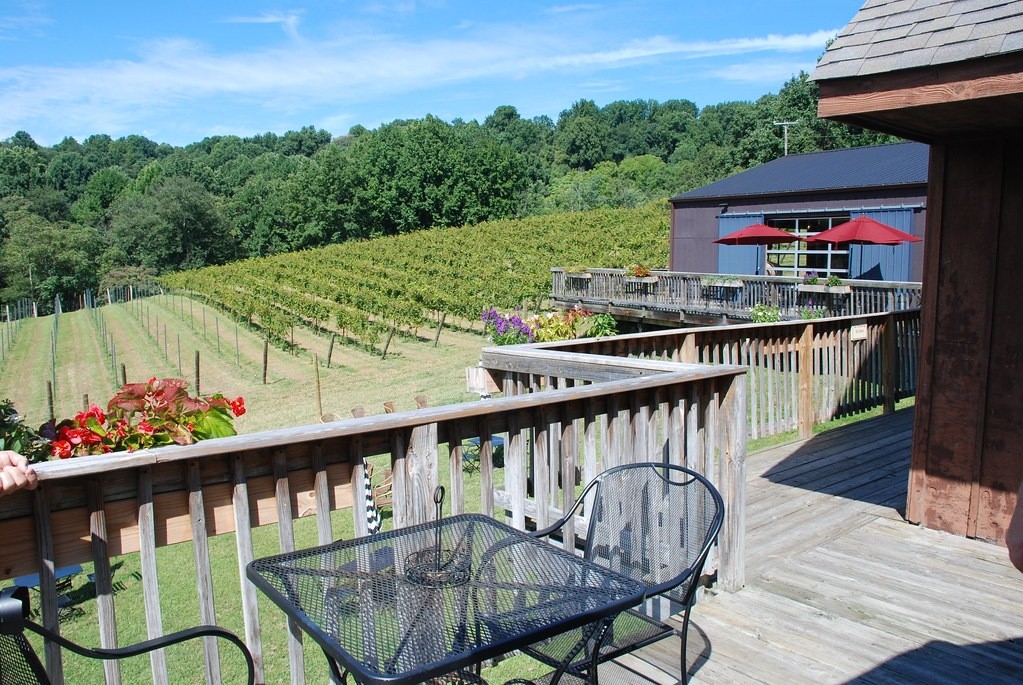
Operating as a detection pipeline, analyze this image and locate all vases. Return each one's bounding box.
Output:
[798,283,850,293]
[0,423,363,583]
[623,276,659,284]
[464,365,545,395]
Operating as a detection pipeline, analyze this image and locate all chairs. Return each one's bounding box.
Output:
[448,444,506,477]
[318,394,427,424]
[57,594,75,618]
[472,460,725,685]
[311,562,397,620]
[87,564,114,583]
[0,586,255,685]
[366,462,393,508]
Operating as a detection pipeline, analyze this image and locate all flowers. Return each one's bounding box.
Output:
[0,378,247,464]
[802,271,842,287]
[624,261,657,277]
[479,305,620,346]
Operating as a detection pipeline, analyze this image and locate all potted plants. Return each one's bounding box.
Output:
[562,263,592,278]
[700,274,745,287]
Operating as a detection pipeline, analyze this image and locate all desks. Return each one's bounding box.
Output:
[245,512,645,685]
[15,564,83,592]
[469,437,504,467]
[339,545,394,575]
